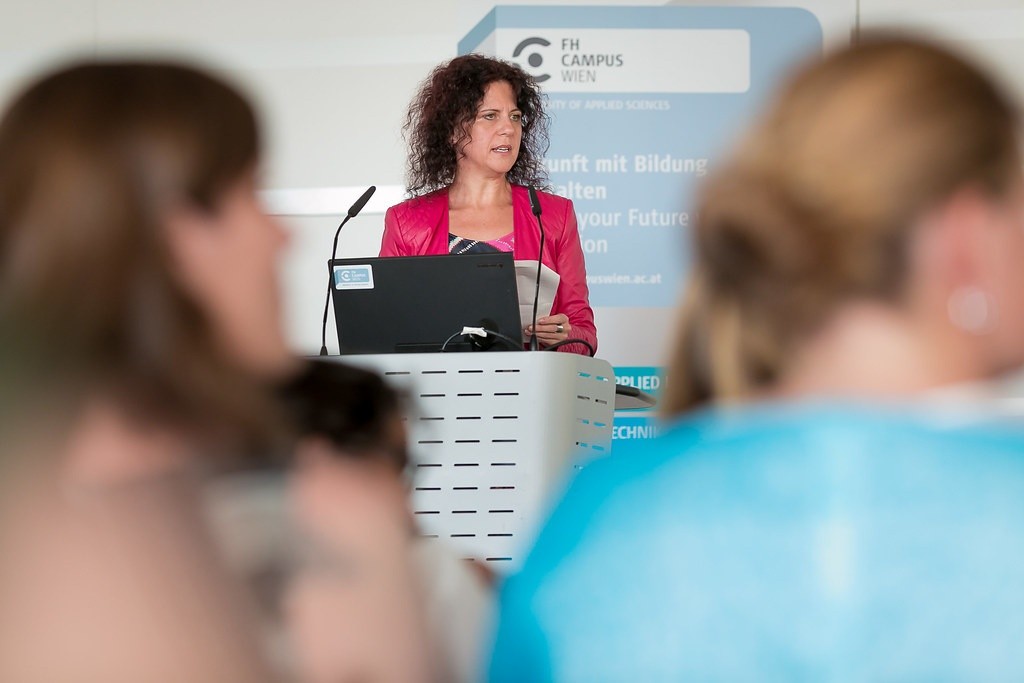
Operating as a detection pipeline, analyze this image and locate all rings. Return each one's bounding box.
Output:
[556,324,563,333]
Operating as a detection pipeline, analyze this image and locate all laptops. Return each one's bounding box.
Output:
[325,250,525,355]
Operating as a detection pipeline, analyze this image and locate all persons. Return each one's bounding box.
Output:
[378,53,598,357]
[0,29,1024,683]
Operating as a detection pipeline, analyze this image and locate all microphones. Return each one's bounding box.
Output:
[319,185,377,354]
[526,184,544,351]
